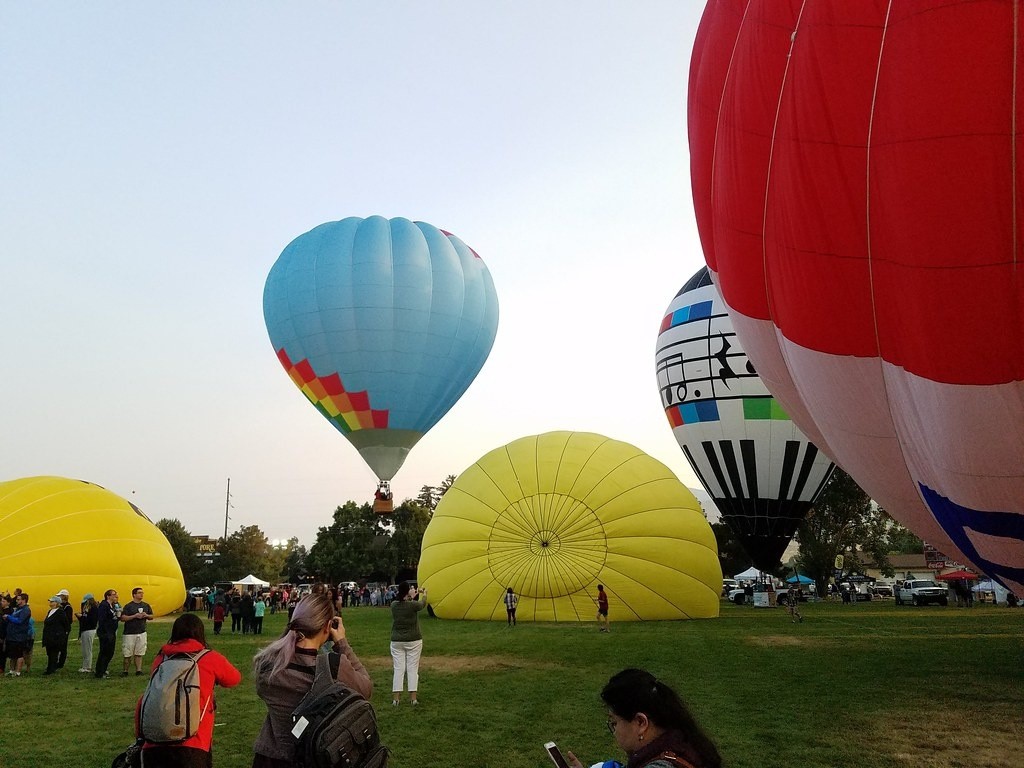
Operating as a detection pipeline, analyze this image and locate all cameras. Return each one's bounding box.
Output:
[417,588,425,593]
[328,619,338,641]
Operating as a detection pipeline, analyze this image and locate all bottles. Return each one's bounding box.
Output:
[589,760,623,768]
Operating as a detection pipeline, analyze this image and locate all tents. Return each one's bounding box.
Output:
[732,567,786,587]
[232,574,270,587]
[838,568,876,597]
[784,571,816,585]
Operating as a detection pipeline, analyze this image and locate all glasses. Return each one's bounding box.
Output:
[607,717,630,734]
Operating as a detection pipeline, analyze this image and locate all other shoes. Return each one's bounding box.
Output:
[119,672,128,677]
[411,699,418,706]
[6,670,20,676]
[600,628,606,631]
[58,663,64,669]
[78,668,91,673]
[393,699,399,706]
[43,670,53,676]
[135,671,143,676]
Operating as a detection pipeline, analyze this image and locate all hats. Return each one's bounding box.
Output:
[55,590,69,596]
[48,596,62,603]
[82,593,93,603]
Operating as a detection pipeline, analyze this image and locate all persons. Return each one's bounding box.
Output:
[131,613,243,768]
[953,583,974,608]
[76,591,100,673]
[390,582,428,707]
[230,588,265,636]
[183,587,229,635]
[252,593,374,768]
[504,588,518,627]
[121,588,155,678]
[94,589,122,679]
[567,669,723,768]
[592,585,609,631]
[906,571,915,580]
[271,573,392,613]
[787,589,802,623]
[376,488,392,500]
[0,589,73,678]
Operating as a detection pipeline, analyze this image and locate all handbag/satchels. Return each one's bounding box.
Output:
[111,741,142,768]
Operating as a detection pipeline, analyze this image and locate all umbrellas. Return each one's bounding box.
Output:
[936,567,982,581]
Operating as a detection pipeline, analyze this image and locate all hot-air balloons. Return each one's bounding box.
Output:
[689,0,1024,603]
[417,431,723,622]
[655,264,838,585]
[2,475,187,619]
[261,215,499,514]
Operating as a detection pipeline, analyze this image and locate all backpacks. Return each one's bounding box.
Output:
[289,653,392,768]
[140,649,213,744]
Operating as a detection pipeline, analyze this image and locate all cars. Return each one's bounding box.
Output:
[261,581,402,607]
[188,586,210,597]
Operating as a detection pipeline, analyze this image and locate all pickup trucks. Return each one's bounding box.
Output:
[867,580,892,597]
[721,578,798,607]
[898,579,948,606]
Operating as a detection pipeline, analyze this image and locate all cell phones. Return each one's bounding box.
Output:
[544,742,571,768]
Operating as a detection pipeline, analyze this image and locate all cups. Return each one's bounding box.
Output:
[115,604,119,608]
[116,611,121,617]
[138,608,143,613]
